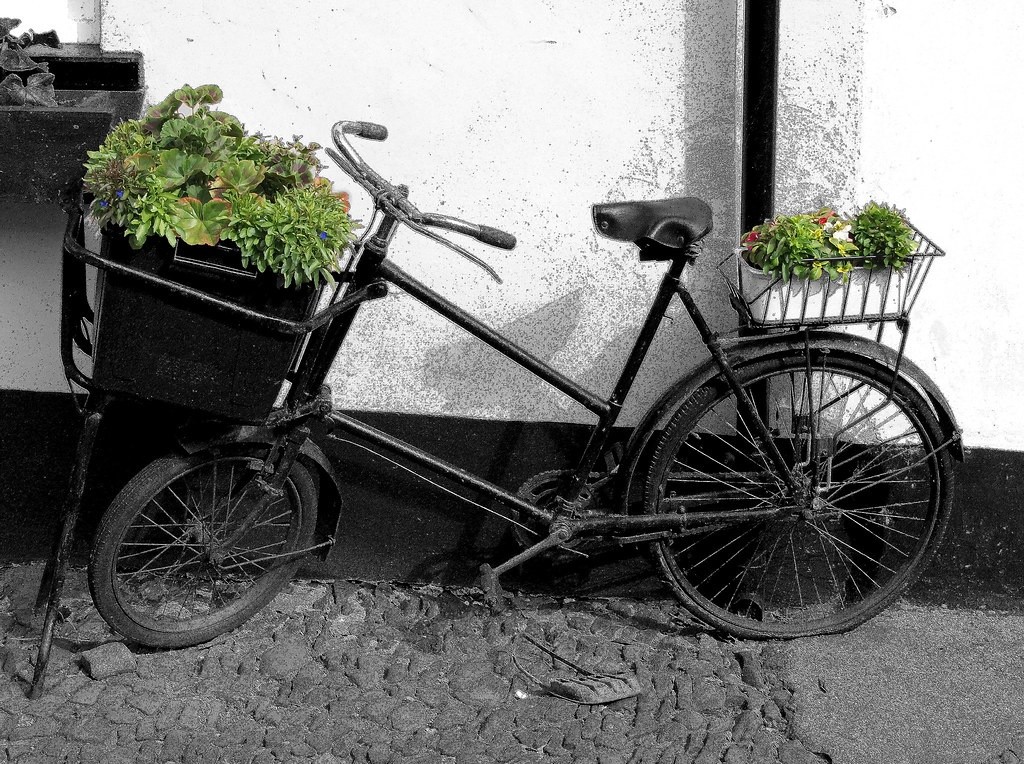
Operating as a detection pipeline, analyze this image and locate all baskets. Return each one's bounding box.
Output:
[716,219,945,330]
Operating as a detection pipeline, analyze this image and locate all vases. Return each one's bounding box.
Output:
[737,240,916,324]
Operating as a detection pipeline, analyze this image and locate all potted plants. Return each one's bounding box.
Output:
[0,17,146,202]
[88,81,365,431]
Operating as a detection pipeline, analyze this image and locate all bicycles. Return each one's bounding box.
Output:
[87,119,965,651]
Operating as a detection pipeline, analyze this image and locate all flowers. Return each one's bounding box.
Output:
[738,206,848,268]
